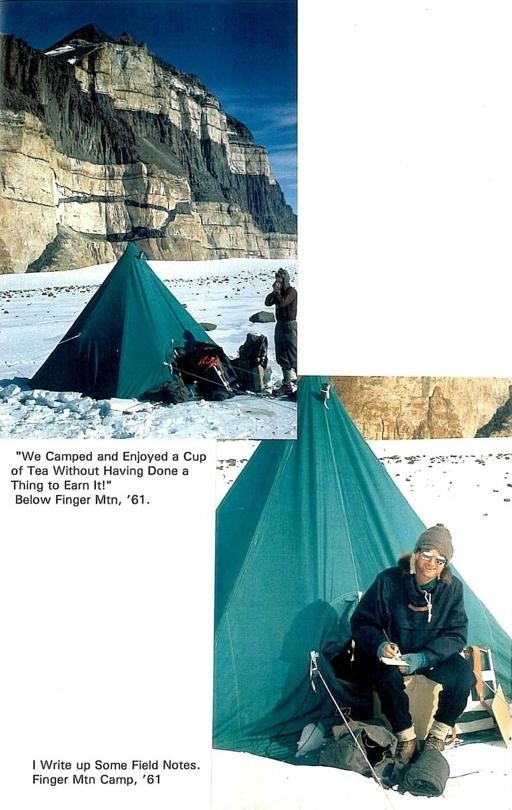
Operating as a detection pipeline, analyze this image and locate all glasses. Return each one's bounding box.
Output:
[421,549,448,566]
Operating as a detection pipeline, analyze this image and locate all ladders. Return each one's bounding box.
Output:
[215,357,242,391]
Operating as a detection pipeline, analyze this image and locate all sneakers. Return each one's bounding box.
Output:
[272,383,293,397]
[393,737,417,763]
[424,731,445,751]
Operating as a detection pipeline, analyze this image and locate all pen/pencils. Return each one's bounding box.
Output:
[382,629,398,660]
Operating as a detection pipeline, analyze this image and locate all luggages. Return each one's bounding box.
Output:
[242,363,267,392]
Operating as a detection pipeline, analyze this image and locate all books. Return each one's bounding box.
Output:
[379,656,410,666]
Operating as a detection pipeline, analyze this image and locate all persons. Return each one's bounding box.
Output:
[264,267,297,402]
[347,522,476,771]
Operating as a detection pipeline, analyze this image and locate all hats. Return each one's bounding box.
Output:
[276,267,290,290]
[414,523,453,560]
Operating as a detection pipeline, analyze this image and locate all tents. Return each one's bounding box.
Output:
[29,242,221,402]
[213,374,511,770]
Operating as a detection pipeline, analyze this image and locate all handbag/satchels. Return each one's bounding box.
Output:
[321,717,398,777]
[241,334,268,358]
